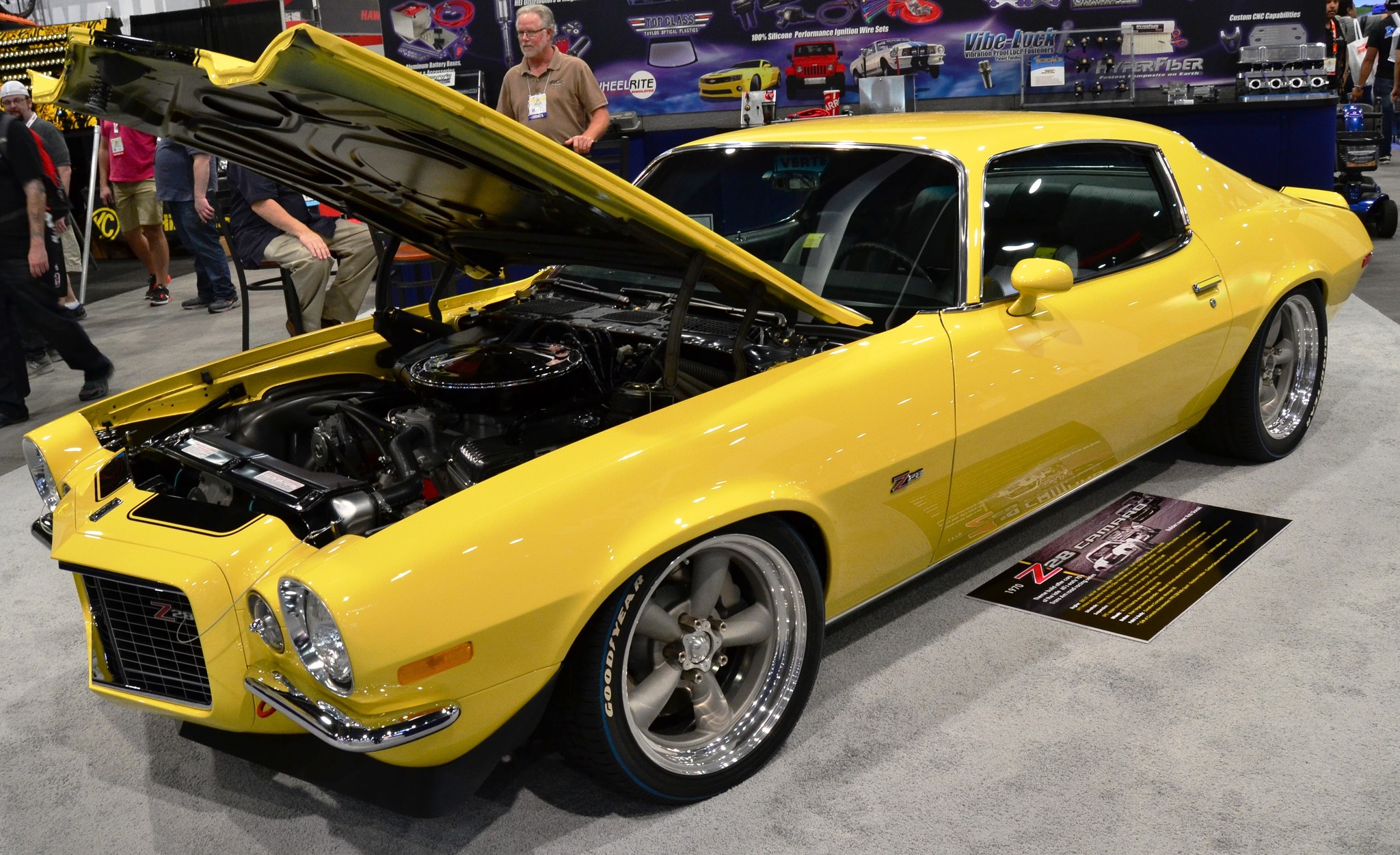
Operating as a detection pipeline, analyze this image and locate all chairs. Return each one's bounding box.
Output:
[562,136,630,183]
[205,186,344,352]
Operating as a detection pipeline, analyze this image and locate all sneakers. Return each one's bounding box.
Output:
[64,304,87,320]
[144,274,170,298]
[208,297,241,312]
[0,403,29,428]
[182,295,211,309]
[79,357,115,400]
[149,284,171,305]
[26,348,62,380]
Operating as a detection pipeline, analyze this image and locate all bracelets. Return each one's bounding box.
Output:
[1344,69,1350,76]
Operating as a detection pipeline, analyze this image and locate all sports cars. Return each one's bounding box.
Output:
[697,57,782,99]
[20,11,1377,829]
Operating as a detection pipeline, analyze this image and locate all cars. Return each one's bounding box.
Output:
[1084,495,1167,575]
[849,36,947,88]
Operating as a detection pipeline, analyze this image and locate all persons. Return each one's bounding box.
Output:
[1390,33,1400,106]
[1351,0,1400,164]
[494,4,610,155]
[97,117,173,305]
[154,134,239,313]
[1338,0,1388,105]
[1326,0,1351,103]
[25,125,70,378]
[0,110,115,427]
[0,81,88,321]
[226,160,385,338]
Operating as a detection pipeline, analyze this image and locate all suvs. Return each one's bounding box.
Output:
[784,39,846,101]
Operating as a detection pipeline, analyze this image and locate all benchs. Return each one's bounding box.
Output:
[902,181,1170,291]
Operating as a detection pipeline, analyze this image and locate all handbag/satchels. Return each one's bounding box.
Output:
[1347,18,1378,86]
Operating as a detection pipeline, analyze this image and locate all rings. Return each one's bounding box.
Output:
[583,145,587,147]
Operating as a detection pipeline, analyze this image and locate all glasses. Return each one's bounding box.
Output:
[514,27,547,38]
[2,97,27,106]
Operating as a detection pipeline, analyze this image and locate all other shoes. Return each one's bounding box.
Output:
[285,318,303,336]
[321,318,341,329]
[1380,156,1391,163]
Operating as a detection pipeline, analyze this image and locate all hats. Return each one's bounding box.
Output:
[1,81,29,98]
[1385,0,1400,5]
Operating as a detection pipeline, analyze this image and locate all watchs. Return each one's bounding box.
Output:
[1355,85,1365,91]
